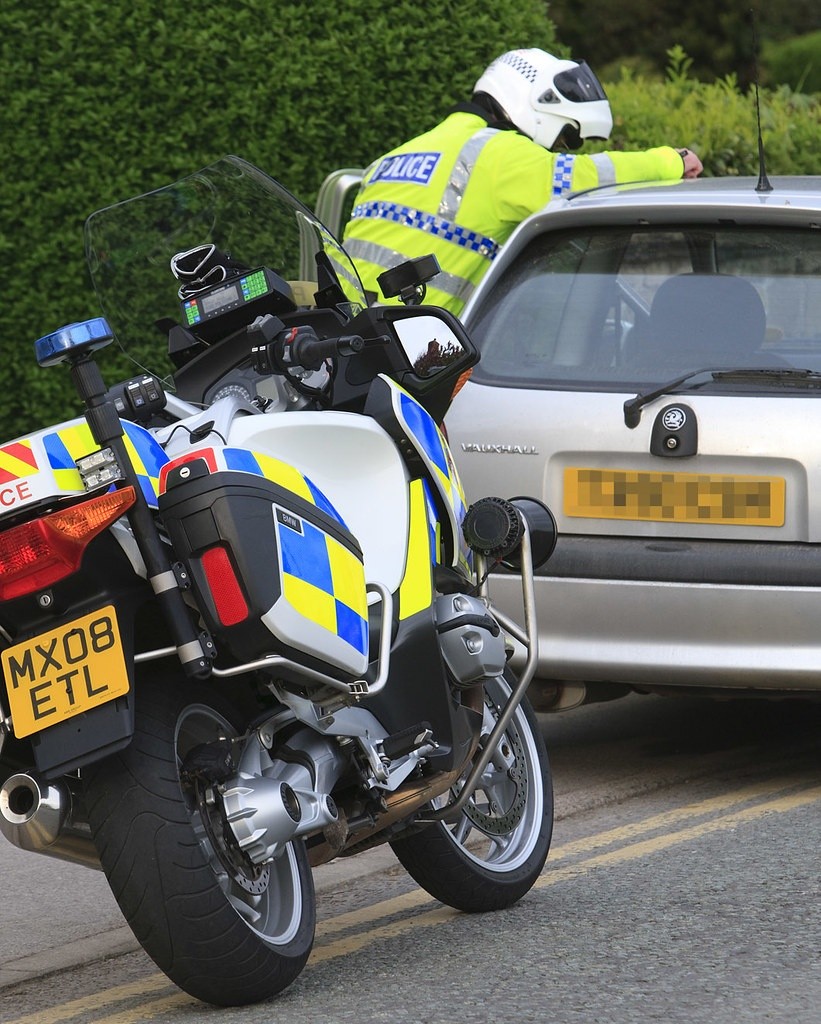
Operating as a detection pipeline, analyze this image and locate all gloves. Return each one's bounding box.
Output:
[177,264,244,300]
[171,244,250,283]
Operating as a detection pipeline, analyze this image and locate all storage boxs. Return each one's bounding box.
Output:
[0,417,171,638]
[156,445,369,697]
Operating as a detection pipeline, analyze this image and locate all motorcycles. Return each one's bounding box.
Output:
[0,152,564,1009]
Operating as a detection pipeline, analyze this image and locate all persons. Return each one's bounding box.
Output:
[340,48,702,326]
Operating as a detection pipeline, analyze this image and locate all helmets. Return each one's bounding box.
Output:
[473,48,613,149]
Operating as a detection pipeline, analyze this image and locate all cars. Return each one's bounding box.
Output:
[312,161,820,712]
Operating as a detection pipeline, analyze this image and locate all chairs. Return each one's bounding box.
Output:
[622,272,790,371]
